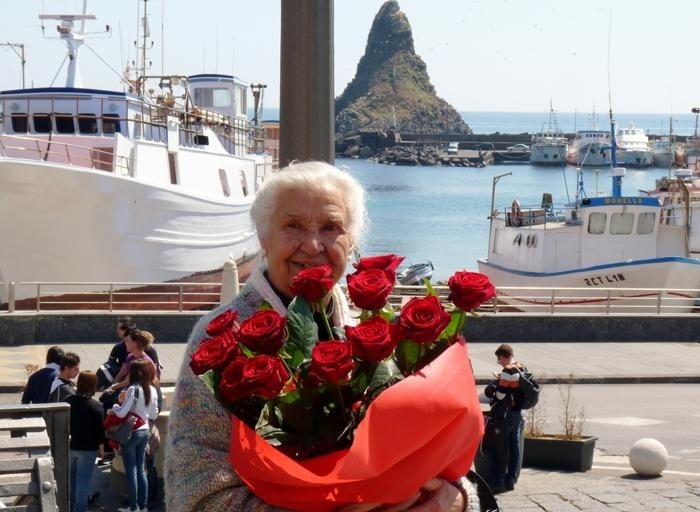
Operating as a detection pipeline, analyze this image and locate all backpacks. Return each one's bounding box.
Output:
[512,361,540,410]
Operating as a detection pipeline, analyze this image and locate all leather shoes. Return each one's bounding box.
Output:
[494,487,514,493]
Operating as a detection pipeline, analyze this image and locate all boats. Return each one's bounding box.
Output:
[529,91,700,170]
[477,106,700,313]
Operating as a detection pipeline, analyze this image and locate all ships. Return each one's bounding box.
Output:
[0,1,279,314]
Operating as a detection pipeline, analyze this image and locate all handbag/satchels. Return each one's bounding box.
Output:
[103,411,145,449]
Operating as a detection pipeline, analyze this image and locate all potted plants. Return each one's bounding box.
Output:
[522,371,598,472]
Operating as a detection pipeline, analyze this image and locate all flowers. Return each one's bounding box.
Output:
[189,252,497,461]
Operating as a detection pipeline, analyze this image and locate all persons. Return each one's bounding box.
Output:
[483,340,525,495]
[20,315,162,511]
[159,158,482,512]
